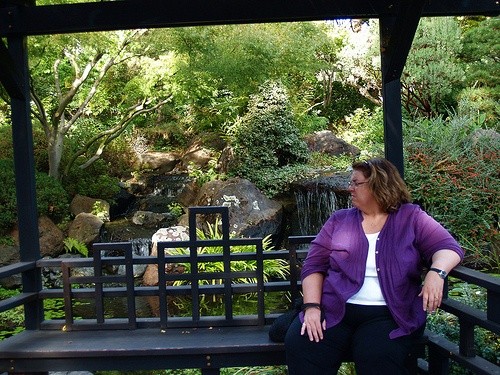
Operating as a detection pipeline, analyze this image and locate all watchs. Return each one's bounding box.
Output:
[427,268,448,279]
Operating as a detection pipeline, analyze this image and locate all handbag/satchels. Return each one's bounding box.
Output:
[269,296,325,342]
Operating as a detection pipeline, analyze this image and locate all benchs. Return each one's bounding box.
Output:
[0,329,287,373]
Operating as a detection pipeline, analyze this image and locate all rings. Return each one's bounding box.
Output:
[434,296,439,298]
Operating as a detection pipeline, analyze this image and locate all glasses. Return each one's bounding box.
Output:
[348,181,369,188]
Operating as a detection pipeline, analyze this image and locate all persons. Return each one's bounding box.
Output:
[283,157,465,374]
